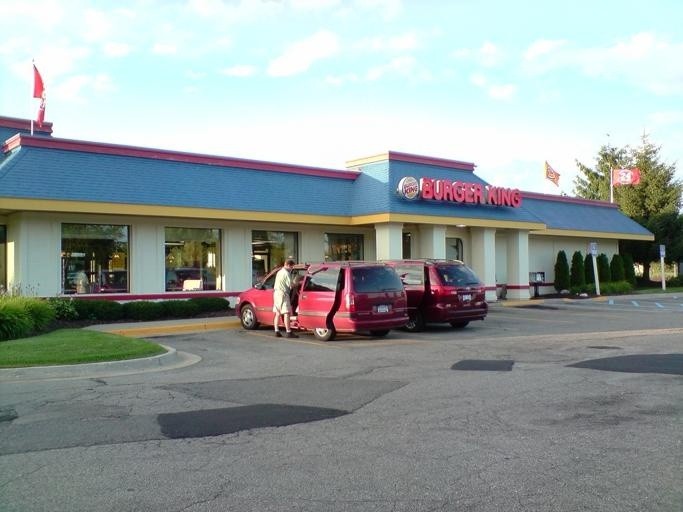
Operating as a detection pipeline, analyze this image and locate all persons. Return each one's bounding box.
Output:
[72,262,89,294]
[271,259,299,339]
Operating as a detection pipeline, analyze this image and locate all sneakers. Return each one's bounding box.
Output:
[275,331,282,337]
[286,332,299,338]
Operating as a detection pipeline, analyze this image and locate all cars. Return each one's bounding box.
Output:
[67,273,89,289]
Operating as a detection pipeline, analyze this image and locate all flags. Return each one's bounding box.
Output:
[32,63,45,99]
[35,99,47,129]
[544,160,559,188]
[610,167,639,188]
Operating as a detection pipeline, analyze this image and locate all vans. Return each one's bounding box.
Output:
[379,259,488,331]
[94,270,128,293]
[168,269,217,290]
[236,259,412,342]
